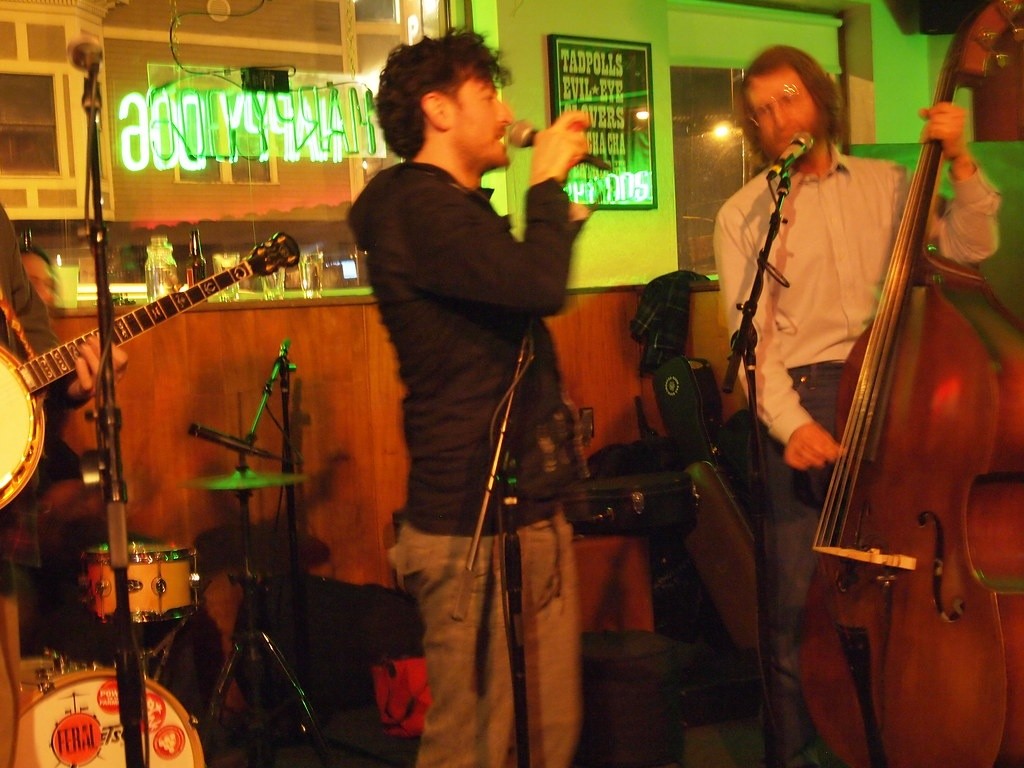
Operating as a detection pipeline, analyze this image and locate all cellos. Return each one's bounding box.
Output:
[795,0,1024,767]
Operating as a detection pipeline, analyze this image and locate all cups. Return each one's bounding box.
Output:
[297,252,324,299]
[51,268,79,310]
[213,255,240,302]
[260,268,284,301]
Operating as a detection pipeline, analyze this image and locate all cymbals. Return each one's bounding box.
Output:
[173,466,307,490]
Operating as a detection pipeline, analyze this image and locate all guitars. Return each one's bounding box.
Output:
[0,229,302,515]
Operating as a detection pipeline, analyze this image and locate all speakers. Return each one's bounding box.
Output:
[919,0,986,35]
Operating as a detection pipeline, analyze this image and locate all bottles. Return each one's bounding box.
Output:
[146,235,178,304]
[186,228,206,290]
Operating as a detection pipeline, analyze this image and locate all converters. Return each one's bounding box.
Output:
[239,68,290,92]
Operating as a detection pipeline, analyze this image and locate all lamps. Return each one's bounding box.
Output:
[240,67,290,94]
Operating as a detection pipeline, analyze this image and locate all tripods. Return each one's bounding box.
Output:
[205,497,341,768]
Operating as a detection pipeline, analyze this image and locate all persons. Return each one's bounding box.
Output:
[348,31,592,768]
[712,44,1000,768]
[0,202,128,665]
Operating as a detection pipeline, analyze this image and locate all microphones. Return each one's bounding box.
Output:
[66,38,103,70]
[766,132,814,180]
[189,424,273,458]
[508,120,611,171]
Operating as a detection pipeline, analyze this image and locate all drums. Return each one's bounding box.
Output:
[77,541,199,624]
[14,657,206,768]
